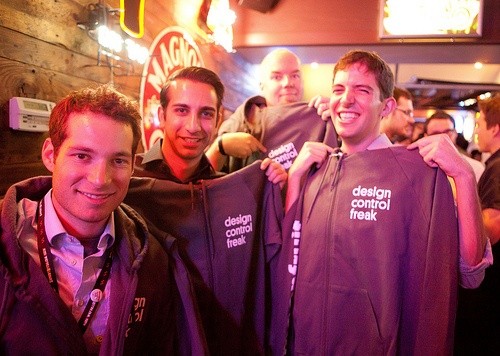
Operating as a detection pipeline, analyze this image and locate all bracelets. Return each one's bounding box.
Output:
[217,132,227,154]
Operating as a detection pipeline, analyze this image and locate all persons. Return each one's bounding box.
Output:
[129,47,500,356]
[0,86,208,356]
